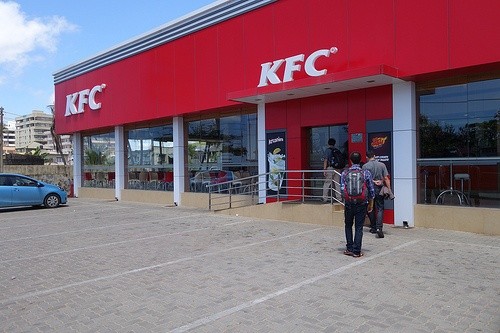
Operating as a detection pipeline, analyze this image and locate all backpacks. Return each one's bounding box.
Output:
[344,169,367,203]
[328,147,346,168]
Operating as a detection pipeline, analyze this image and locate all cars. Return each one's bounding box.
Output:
[189,169,234,190]
[0,172,67,210]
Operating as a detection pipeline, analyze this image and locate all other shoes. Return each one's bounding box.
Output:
[369,227,377,233]
[377,227,384,238]
[344,249,364,258]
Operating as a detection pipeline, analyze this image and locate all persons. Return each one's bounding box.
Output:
[341,152,375,257]
[421,148,442,204]
[320,138,342,202]
[362,150,391,238]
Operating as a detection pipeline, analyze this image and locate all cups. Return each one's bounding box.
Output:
[268,154,286,191]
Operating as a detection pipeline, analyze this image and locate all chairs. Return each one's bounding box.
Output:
[128,168,173,191]
[188,168,258,194]
[83,169,115,189]
